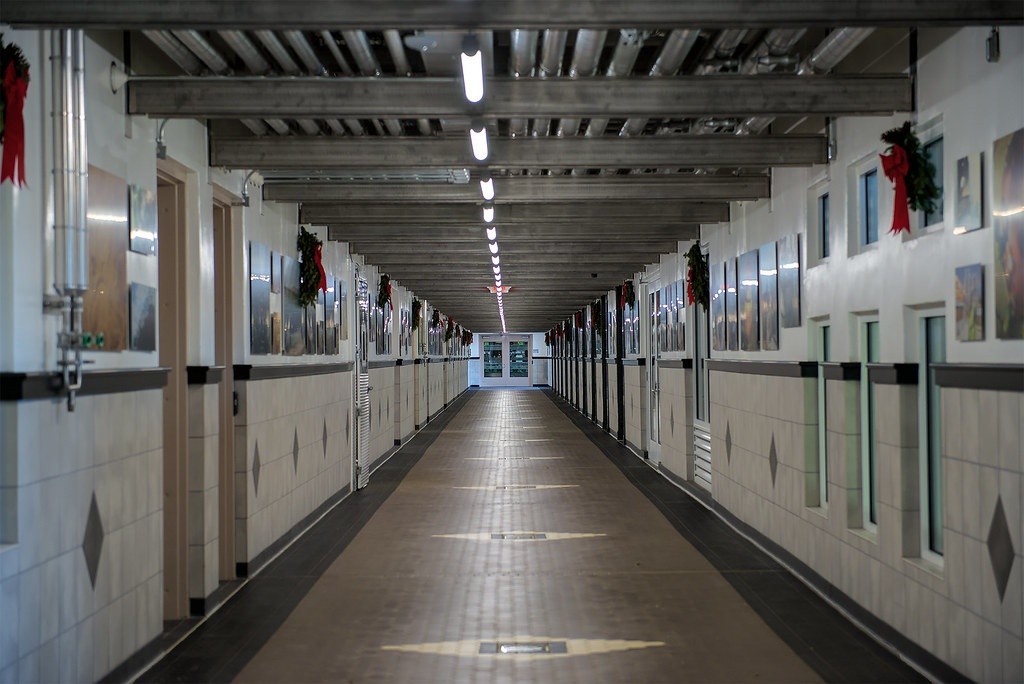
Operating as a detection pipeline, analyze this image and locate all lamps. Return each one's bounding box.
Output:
[459,34,503,306]
